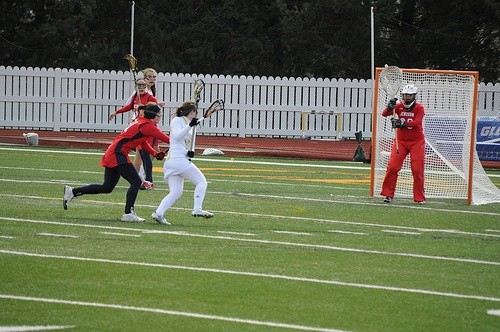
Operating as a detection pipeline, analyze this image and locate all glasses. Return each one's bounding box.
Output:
[138,83,146,86]
[156,114,161,118]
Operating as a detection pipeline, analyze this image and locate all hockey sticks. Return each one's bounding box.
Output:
[187,78,206,158]
[123,54,142,106]
[379,65,403,154]
[156,99,225,160]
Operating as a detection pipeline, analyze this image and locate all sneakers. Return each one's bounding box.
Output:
[384,195,391,202]
[63,186,75,209]
[140,181,154,189]
[151,212,172,226]
[191,209,214,218]
[121,207,146,222]
[418,201,426,205]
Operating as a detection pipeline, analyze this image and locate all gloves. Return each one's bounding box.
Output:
[391,118,406,128]
[156,151,165,160]
[188,151,194,158]
[189,117,200,127]
[387,97,398,110]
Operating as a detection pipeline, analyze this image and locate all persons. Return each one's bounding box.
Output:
[109,68,165,190]
[63,105,170,222]
[380,85,426,205]
[151,100,214,226]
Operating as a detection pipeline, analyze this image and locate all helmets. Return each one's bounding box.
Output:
[401,85,417,109]
